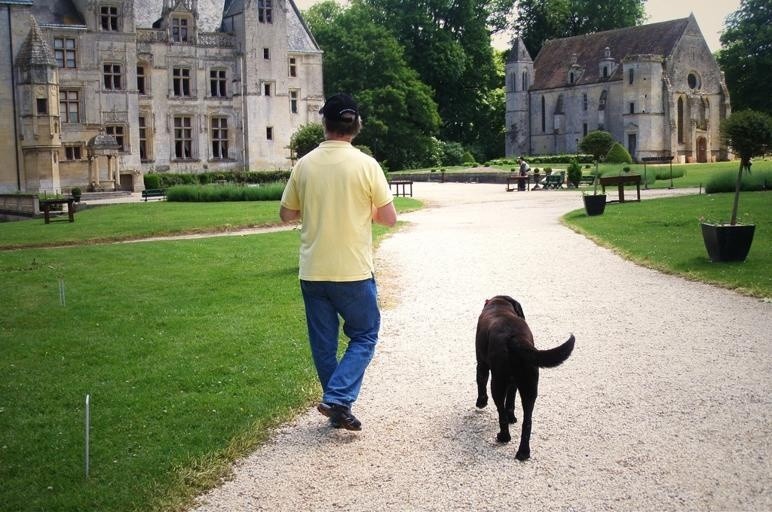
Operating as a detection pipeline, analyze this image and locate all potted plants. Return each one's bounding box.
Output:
[578,130,614,216]
[700,108,772,263]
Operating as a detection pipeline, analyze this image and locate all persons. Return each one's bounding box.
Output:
[515,156,529,191]
[276,93,399,433]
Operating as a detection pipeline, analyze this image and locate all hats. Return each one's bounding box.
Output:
[319,96,360,122]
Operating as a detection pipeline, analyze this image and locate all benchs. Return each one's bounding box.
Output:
[141,188,167,202]
[531,175,597,190]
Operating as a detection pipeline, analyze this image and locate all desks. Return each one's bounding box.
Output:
[388,181,413,197]
[600,175,642,203]
[506,176,530,191]
[40,198,76,224]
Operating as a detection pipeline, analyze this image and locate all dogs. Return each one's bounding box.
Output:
[474,294,577,461]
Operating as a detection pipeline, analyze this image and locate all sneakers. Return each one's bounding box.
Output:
[318,402,362,431]
